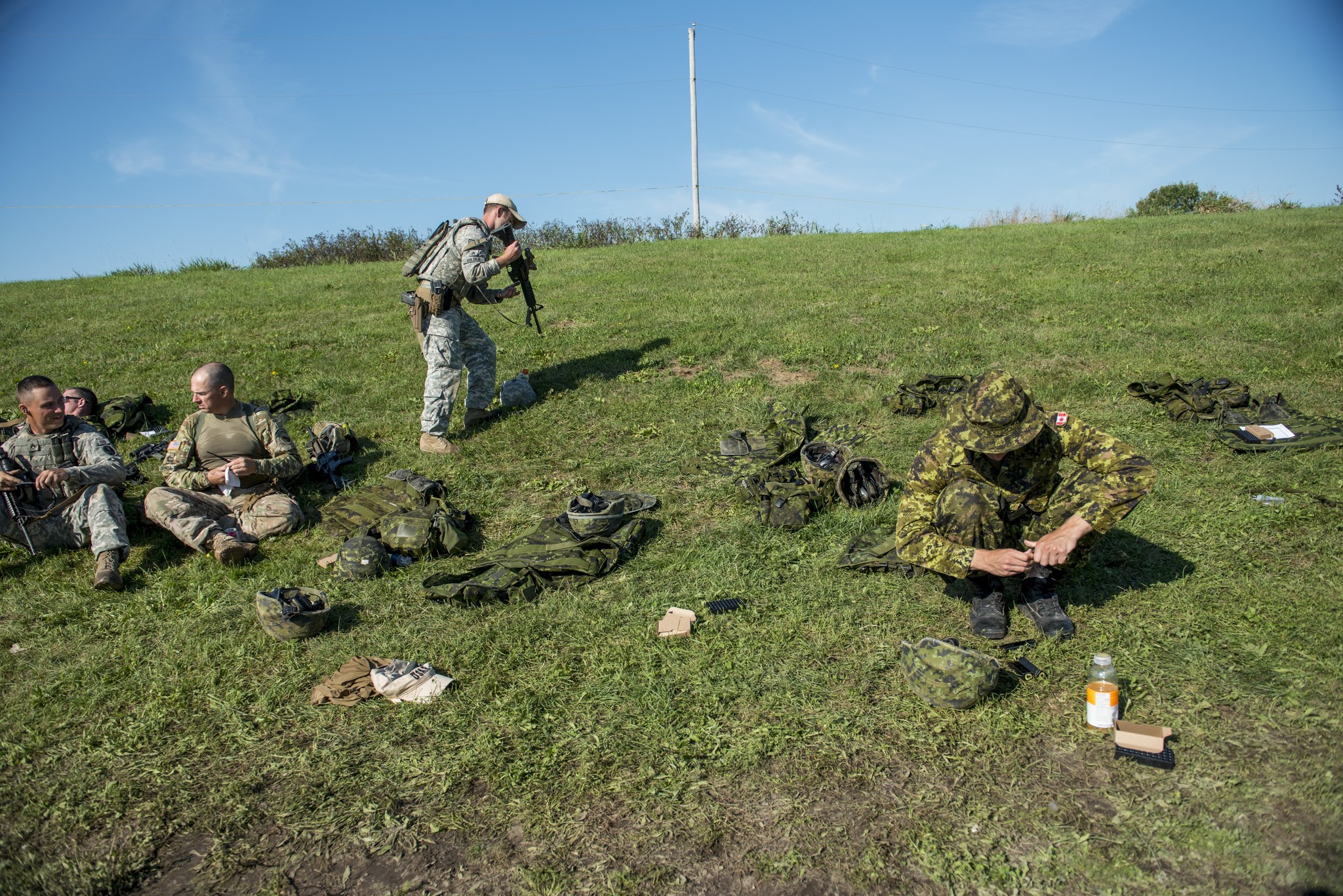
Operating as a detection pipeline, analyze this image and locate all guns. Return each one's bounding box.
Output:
[401,292,423,332]
[490,222,544,334]
[305,425,358,491]
[124,426,179,481]
[0,446,57,557]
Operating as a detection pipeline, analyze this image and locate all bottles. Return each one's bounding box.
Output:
[1086,652,1119,734]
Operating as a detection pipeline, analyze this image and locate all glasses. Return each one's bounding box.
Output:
[62,396,89,404]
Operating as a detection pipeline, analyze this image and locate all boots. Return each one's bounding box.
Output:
[93,549,123,591]
[420,432,462,456]
[464,407,502,429]
[212,533,259,567]
[964,572,1009,639]
[1017,562,1076,640]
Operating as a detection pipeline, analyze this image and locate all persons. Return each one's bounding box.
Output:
[894,368,1158,639]
[0,374,133,591]
[145,362,303,567]
[401,193,527,456]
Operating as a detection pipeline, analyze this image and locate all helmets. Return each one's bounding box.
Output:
[800,440,854,486]
[256,587,331,642]
[317,423,350,460]
[567,489,625,537]
[333,535,393,579]
[499,378,537,407]
[901,635,1000,711]
[836,456,890,509]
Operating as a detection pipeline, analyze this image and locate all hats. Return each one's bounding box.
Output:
[598,490,657,514]
[484,193,527,229]
[311,421,360,454]
[947,370,1046,454]
